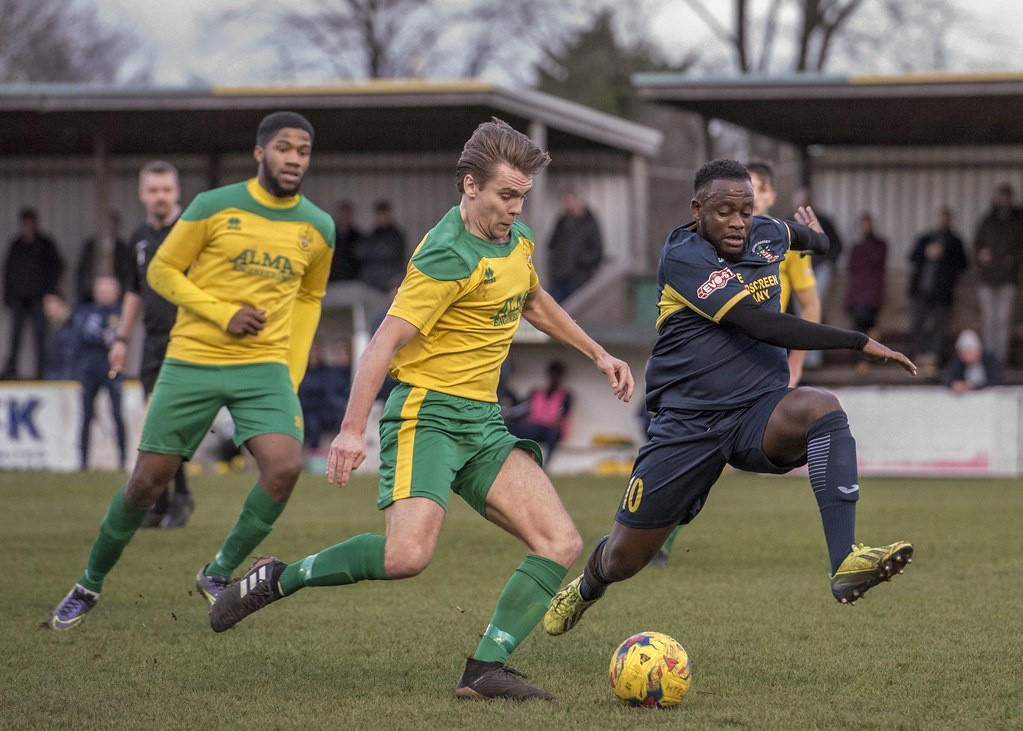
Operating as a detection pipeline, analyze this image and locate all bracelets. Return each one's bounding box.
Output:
[114,335,127,342]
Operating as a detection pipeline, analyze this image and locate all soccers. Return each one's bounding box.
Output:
[608,631,692,708]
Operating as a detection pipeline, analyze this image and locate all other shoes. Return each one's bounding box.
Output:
[143,493,170,529]
[160,493,195,528]
[648,550,668,568]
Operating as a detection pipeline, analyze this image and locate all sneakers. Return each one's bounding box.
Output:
[455,656,556,703]
[544,573,606,636]
[211,554,289,634]
[830,539,913,606]
[51,583,101,632]
[195,563,230,609]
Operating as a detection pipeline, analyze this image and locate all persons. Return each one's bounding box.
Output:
[842,213,888,374]
[976,183,1023,364]
[76,207,129,309]
[106,160,196,528]
[42,276,127,474]
[297,335,352,451]
[943,328,1004,392]
[0,208,62,380]
[651,159,822,565]
[51,111,335,630]
[510,358,572,447]
[208,116,634,702]
[547,184,603,304]
[543,159,918,637]
[906,207,969,357]
[328,197,406,293]
[785,185,843,370]
